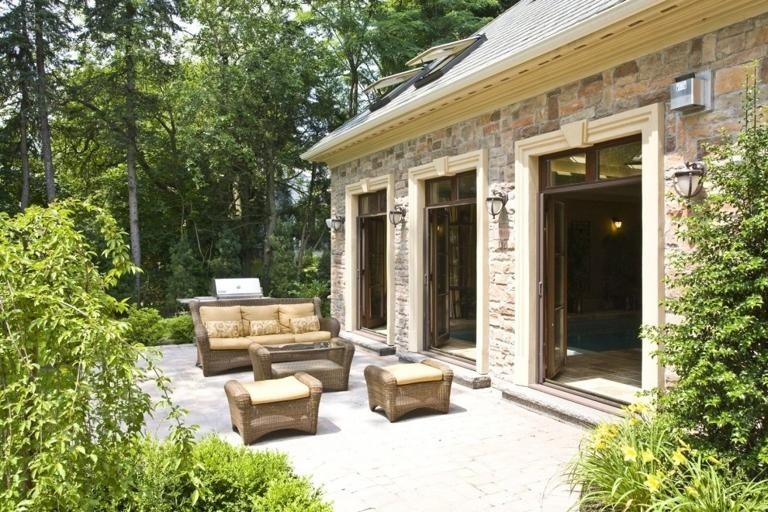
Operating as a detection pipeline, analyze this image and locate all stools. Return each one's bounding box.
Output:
[223,357,455,448]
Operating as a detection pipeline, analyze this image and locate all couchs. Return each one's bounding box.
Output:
[188,295,342,377]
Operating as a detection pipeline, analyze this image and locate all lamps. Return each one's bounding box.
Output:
[671,160,705,209]
[388,203,407,229]
[484,187,509,220]
[330,214,346,232]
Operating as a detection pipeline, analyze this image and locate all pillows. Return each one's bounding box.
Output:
[200,315,322,339]
